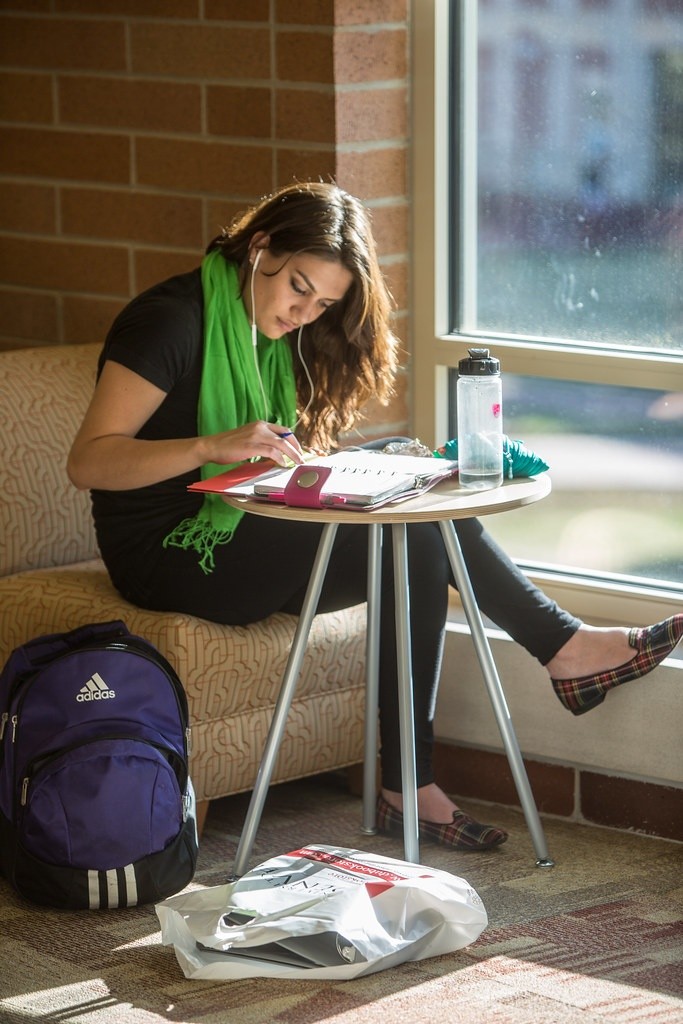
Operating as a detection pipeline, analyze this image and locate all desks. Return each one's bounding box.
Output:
[219,459,554,882]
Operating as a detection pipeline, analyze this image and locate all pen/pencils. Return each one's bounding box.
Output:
[278,432,293,438]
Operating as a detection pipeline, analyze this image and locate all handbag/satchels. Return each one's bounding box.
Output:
[154,841,489,981]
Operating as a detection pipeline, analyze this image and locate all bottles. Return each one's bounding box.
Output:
[457,348,503,492]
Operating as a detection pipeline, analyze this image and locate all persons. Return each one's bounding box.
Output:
[68,184,683,851]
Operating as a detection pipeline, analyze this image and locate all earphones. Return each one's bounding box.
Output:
[252,248,265,271]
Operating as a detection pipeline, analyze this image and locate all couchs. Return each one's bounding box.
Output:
[0,340,381,837]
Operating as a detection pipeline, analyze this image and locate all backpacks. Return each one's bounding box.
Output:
[0,618,198,913]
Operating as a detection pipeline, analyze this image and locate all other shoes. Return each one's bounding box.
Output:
[375,788,509,850]
[549,614,683,716]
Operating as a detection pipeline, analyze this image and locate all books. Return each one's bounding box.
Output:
[247,450,458,512]
